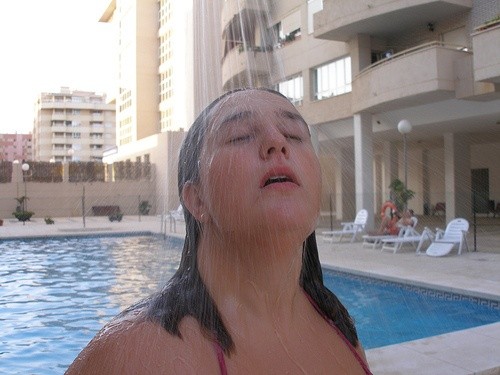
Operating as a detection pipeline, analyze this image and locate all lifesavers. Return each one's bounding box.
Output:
[380,201,398,227]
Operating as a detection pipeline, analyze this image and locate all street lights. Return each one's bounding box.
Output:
[21,162,30,213]
[397,119,413,214]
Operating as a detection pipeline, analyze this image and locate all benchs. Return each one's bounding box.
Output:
[380,225,433,254]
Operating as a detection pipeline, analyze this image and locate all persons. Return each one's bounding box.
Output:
[65,89,373,375]
[367,209,415,235]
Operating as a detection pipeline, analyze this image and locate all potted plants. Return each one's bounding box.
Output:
[12,195,34,222]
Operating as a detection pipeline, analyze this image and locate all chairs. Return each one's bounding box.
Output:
[426,219,470,256]
[362,216,417,248]
[322,210,367,244]
